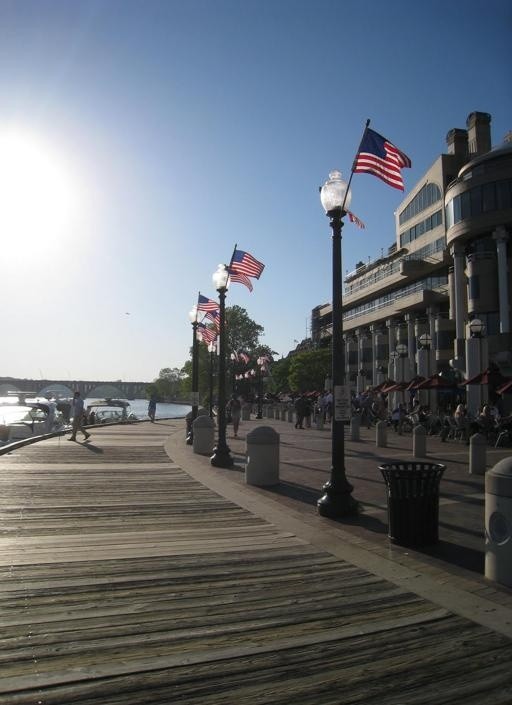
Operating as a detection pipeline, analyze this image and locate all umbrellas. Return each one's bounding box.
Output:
[367,368,511,395]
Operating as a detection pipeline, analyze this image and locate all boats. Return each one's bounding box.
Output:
[0,396,131,448]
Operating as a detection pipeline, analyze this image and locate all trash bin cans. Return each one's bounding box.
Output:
[378,462,447,548]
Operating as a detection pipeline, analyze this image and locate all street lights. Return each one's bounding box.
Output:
[317,171,360,517]
[256,357,264,418]
[185,265,234,468]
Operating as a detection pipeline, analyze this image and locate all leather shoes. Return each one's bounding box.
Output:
[67,433,91,441]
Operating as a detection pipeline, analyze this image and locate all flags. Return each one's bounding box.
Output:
[196,291,221,312]
[239,351,251,366]
[201,335,212,345]
[198,323,218,341]
[348,211,366,231]
[204,309,228,331]
[351,117,413,195]
[227,266,254,293]
[230,243,266,280]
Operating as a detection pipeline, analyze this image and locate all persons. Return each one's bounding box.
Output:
[226,392,241,438]
[290,387,512,449]
[146,394,157,423]
[65,392,91,442]
